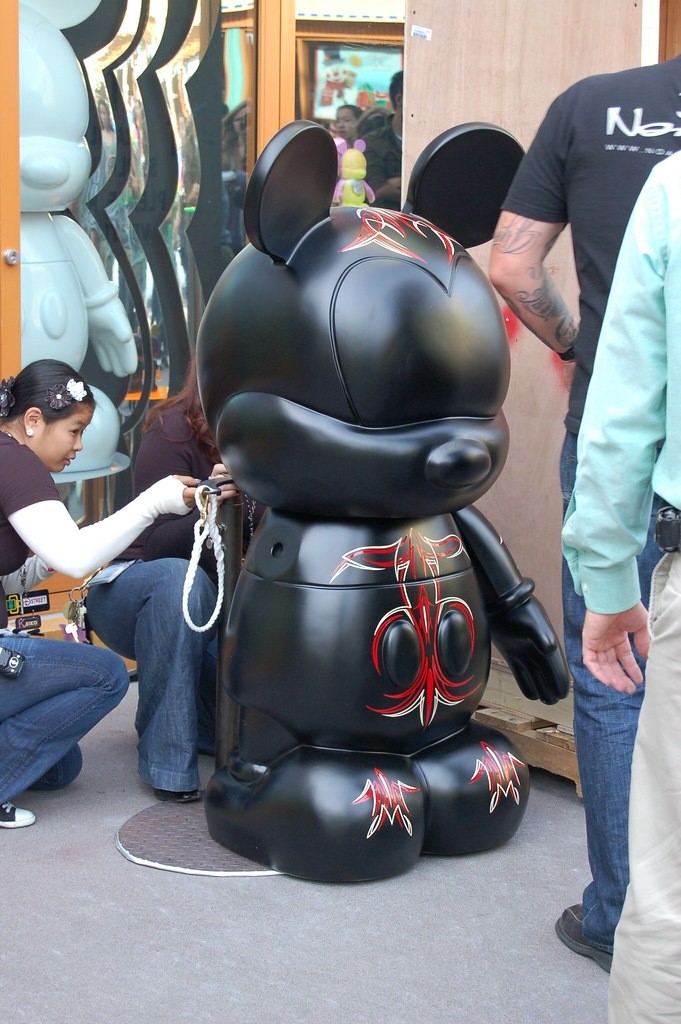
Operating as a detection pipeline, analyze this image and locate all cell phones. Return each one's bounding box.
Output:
[0,646,25,679]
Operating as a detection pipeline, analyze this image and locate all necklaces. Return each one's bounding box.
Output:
[0,430,29,594]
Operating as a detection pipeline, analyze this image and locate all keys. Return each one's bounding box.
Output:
[59,600,87,643]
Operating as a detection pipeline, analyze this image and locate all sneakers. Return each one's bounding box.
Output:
[0,802,35,828]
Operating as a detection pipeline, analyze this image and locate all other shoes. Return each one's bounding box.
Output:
[155,788,201,802]
[555,904,612,972]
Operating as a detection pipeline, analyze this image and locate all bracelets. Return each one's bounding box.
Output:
[558,347,576,363]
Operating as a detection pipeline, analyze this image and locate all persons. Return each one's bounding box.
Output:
[219,100,249,262]
[86,354,267,802]
[336,70,403,211]
[488,54,681,974]
[0,359,202,828]
[562,148,681,1024]
